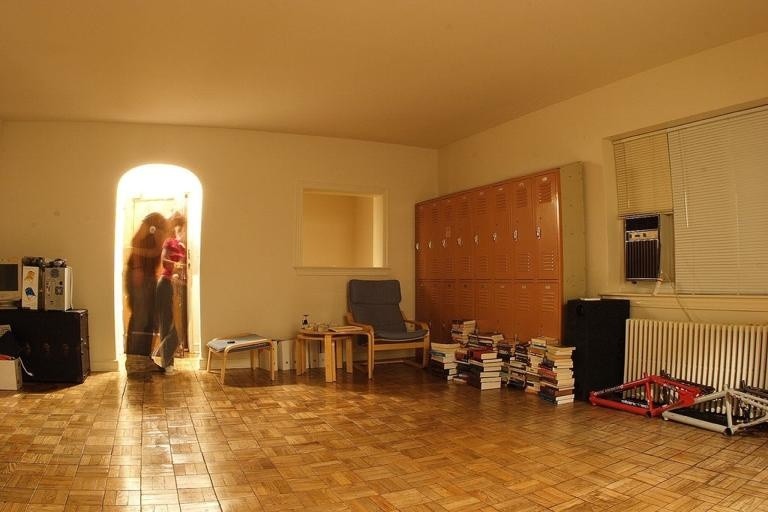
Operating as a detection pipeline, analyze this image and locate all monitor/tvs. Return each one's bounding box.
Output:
[0,255,22,307]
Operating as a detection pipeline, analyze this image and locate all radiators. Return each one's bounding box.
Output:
[624,315,767,419]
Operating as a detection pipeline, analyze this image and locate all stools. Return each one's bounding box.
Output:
[208,332,278,383]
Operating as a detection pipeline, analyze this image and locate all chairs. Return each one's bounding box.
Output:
[345,277,432,380]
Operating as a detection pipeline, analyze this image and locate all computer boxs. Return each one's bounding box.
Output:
[22,265,43,311]
[44,267,72,312]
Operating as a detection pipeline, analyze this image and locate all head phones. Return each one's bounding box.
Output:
[46,259,66,267]
[21,257,45,272]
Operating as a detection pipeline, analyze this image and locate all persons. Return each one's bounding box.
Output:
[155,213,186,374]
[122,214,170,383]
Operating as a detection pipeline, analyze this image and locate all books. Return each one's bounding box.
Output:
[428,318,577,406]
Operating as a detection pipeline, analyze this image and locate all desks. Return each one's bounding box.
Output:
[293,326,374,381]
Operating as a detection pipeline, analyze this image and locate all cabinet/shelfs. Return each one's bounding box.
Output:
[19,307,92,384]
[415,162,586,351]
[566,299,631,397]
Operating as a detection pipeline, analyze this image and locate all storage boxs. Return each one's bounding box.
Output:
[1,354,23,392]
[261,337,327,370]
